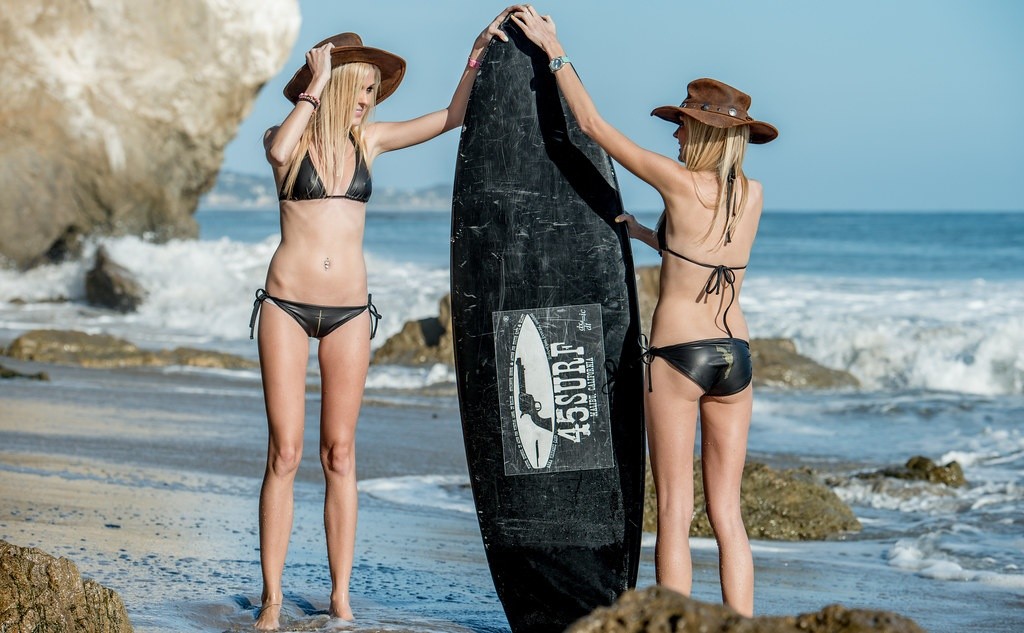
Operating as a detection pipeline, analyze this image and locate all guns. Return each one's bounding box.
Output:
[515,356,554,433]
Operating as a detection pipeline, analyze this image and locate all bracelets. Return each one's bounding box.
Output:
[467,57,479,68]
[297,92,320,113]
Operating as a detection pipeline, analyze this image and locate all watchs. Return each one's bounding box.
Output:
[549,54,571,72]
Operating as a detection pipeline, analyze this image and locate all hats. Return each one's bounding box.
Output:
[650,78,778,144]
[283,32,406,107]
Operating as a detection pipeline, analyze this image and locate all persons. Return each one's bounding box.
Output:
[506,3,778,619]
[248,4,527,629]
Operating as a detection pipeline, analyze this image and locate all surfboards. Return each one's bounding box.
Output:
[450,10,646,633]
